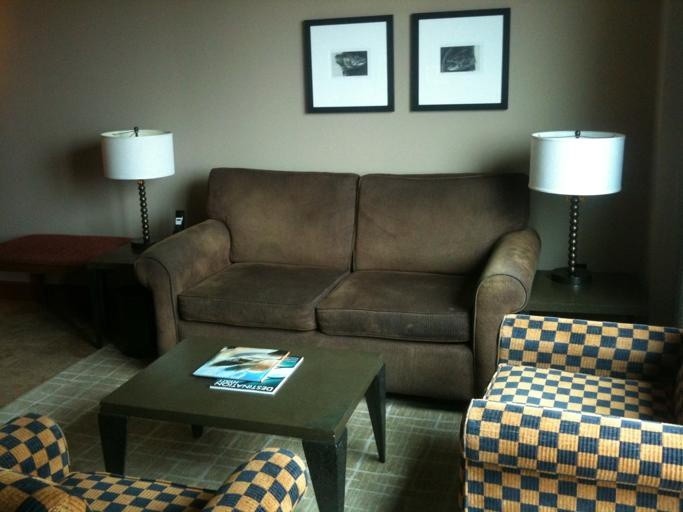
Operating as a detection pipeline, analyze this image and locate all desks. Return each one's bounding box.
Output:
[0,234,133,313]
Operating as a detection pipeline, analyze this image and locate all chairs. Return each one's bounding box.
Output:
[462,312,683,512]
[0,413,308,512]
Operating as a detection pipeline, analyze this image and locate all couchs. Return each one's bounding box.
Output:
[135,167,542,411]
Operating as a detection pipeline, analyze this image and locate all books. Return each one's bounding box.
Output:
[192,345,290,383]
[208,355,304,395]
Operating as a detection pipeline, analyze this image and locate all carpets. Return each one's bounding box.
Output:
[0,341,469,512]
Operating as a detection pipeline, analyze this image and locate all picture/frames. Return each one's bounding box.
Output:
[301,14,394,113]
[410,6,510,111]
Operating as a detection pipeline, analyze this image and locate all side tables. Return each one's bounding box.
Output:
[92,234,163,358]
[523,270,649,320]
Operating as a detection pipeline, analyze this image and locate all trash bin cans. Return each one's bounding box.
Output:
[111,274,156,358]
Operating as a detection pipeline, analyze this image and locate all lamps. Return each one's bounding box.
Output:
[100,127,175,249]
[526,128,625,288]
